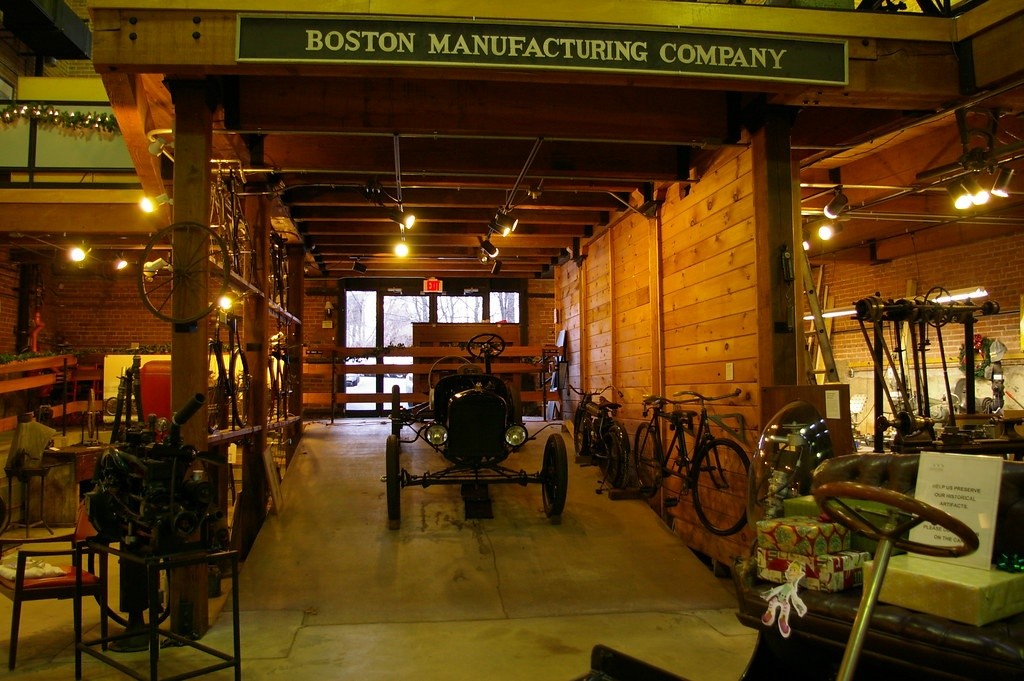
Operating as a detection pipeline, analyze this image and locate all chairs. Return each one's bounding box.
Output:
[0,502,109,671]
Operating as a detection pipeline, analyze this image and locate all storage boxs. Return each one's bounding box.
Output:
[863,554,1024,627]
[754,515,871,592]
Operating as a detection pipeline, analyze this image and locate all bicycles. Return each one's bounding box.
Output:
[207,306,252,433]
[567,383,629,493]
[269,230,290,311]
[633,388,754,536]
[269,336,294,421]
[208,159,254,283]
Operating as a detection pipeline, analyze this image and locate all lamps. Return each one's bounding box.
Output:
[148,136,175,156]
[143,258,173,278]
[948,176,989,209]
[818,223,843,240]
[488,215,518,237]
[73,243,92,261]
[325,301,334,316]
[113,251,128,269]
[477,240,499,265]
[805,285,988,320]
[489,260,502,275]
[823,193,848,220]
[352,261,367,274]
[388,209,414,230]
[139,193,172,213]
[991,167,1015,197]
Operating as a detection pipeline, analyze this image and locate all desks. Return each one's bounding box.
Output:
[28,442,111,528]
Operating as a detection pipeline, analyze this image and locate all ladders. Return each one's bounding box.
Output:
[802,246,839,385]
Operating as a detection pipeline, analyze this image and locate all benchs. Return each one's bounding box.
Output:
[737,452,1024,681]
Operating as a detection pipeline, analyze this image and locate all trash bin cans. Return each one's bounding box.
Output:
[139,361,172,442]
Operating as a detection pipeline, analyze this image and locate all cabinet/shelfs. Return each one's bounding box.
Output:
[411,322,521,415]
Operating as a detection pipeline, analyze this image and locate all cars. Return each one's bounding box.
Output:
[346,373,361,387]
